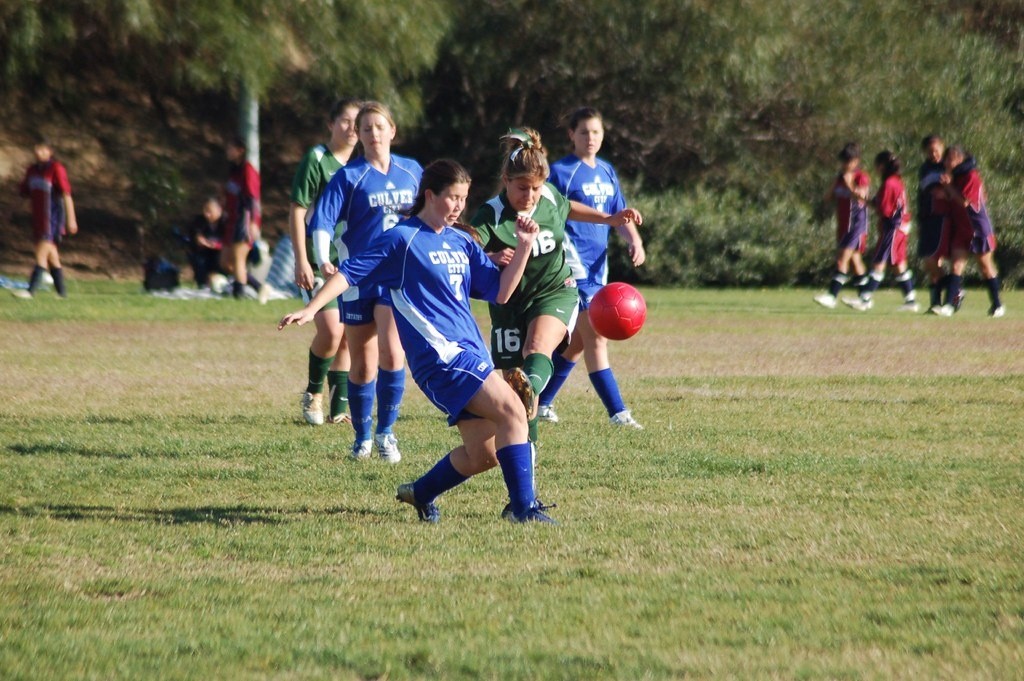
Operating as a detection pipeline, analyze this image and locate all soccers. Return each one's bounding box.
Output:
[587,281,647,341]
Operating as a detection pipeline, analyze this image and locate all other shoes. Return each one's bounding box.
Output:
[841,298,866,312]
[988,305,1003,319]
[929,304,953,317]
[898,302,922,312]
[11,289,32,299]
[813,296,835,309]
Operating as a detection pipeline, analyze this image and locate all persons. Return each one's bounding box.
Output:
[186,197,271,292]
[546,109,647,434]
[465,127,644,489]
[288,98,372,425]
[219,134,262,297]
[841,150,919,312]
[915,135,1006,317]
[815,144,873,309]
[278,158,558,526]
[312,102,427,464]
[265,231,302,297]
[11,135,78,300]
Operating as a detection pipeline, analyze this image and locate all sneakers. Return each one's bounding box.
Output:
[608,411,645,430]
[507,367,537,421]
[327,413,351,424]
[501,499,557,524]
[350,438,373,462]
[302,391,324,426]
[374,434,400,464]
[537,405,559,422]
[394,482,440,523]
